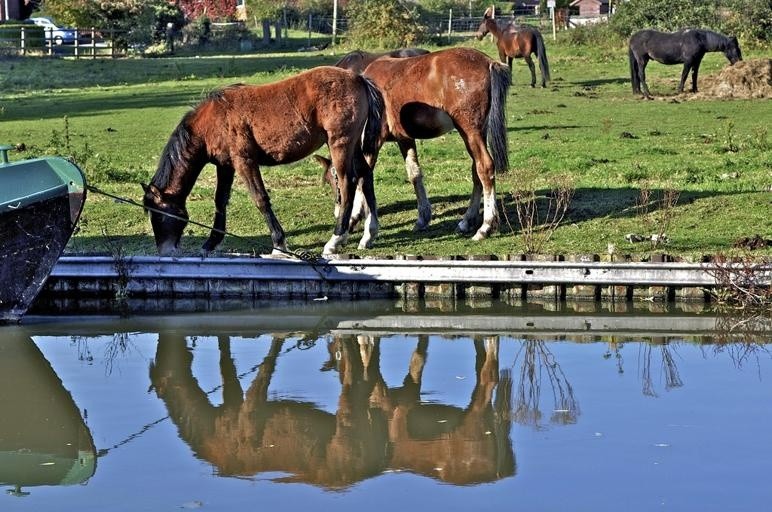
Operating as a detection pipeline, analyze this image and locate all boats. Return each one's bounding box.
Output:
[0,145,87,324]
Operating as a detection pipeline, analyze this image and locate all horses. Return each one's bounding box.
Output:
[312,48,512,241]
[319,336,517,486]
[476,13,550,88]
[147,330,395,491]
[630,28,742,100]
[140,65,385,256]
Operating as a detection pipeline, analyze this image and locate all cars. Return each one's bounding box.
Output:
[23,17,81,46]
